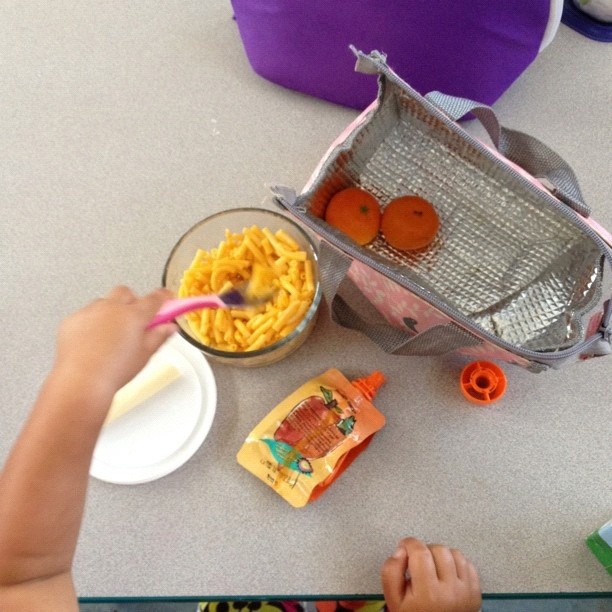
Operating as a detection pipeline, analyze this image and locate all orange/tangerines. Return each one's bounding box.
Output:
[381,195,440,250]
[327,187,379,245]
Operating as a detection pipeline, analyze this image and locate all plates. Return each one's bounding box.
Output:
[87,332,218,487]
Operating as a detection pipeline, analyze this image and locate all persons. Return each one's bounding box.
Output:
[0,284,484,611]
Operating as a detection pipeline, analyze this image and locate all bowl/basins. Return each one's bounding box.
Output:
[160,207,324,367]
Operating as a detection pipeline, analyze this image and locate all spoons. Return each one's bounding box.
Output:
[142,275,270,328]
